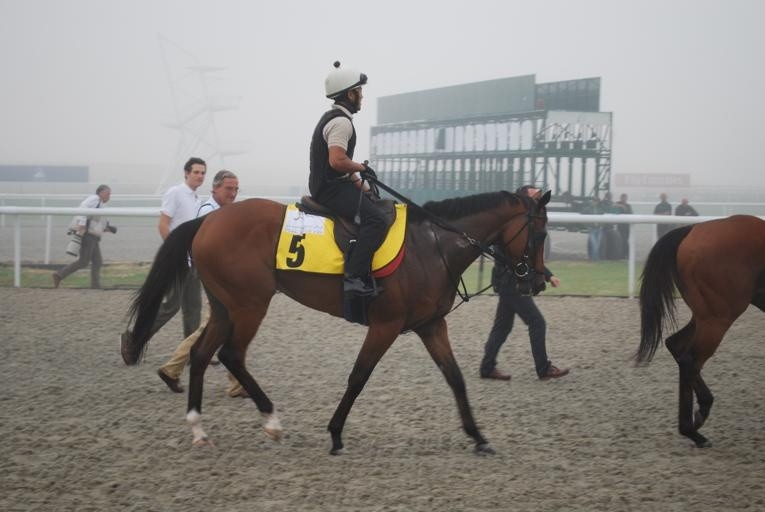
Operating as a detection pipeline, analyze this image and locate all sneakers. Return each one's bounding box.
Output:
[52,273,62,289]
[539,365,570,381]
[157,367,184,393]
[120,331,141,366]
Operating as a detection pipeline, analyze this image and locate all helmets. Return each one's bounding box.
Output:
[325,60,367,98]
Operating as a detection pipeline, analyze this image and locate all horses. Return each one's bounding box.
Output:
[123,185,552,456]
[625,214,765,447]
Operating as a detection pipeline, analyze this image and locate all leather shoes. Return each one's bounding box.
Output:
[481,368,511,380]
[343,277,385,299]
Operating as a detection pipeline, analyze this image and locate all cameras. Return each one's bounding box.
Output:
[104,222,116,233]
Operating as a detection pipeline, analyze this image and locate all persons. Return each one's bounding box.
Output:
[121,157,248,398]
[52,184,117,288]
[479,185,571,381]
[654,192,673,238]
[587,191,633,260]
[674,197,699,216]
[308,67,391,297]
